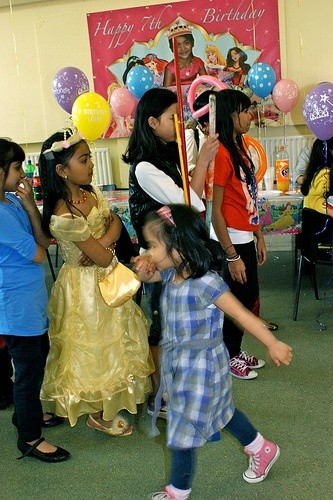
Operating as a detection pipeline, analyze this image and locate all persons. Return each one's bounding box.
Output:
[39,132,156,436]
[163,26,207,87]
[0,137,70,462]
[209,88,268,379]
[132,204,294,500]
[122,89,219,412]
[184,90,219,199]
[294,136,333,265]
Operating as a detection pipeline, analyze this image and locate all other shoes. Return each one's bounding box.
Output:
[147,393,167,420]
[257,316,278,331]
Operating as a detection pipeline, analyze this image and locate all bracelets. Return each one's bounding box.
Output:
[224,244,241,262]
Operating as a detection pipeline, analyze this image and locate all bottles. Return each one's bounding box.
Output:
[276,146,290,194]
[33,163,46,201]
[23,160,35,187]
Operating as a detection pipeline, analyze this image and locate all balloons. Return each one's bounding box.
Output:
[72,92,111,141]
[110,87,136,117]
[303,82,333,140]
[272,80,300,113]
[127,65,154,99]
[248,62,277,98]
[186,75,228,119]
[52,67,89,114]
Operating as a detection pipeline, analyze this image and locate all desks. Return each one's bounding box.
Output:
[37,189,305,291]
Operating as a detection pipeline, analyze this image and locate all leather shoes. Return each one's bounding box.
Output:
[42,412,65,428]
[17,437,70,462]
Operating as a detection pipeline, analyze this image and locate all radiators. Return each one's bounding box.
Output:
[252,131,317,183]
[22,149,113,186]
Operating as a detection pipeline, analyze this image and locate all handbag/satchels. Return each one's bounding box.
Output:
[98,248,141,308]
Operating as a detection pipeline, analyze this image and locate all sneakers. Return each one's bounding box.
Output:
[238,351,265,368]
[242,440,280,483]
[148,486,191,500]
[230,355,258,379]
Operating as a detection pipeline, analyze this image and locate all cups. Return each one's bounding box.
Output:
[263,166,275,192]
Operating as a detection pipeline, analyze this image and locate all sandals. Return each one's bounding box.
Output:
[86,414,133,436]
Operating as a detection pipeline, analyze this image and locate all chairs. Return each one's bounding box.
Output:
[291,229,333,322]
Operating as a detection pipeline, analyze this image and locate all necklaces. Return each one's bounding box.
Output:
[68,188,86,205]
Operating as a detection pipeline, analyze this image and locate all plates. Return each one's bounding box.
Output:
[257,190,282,198]
